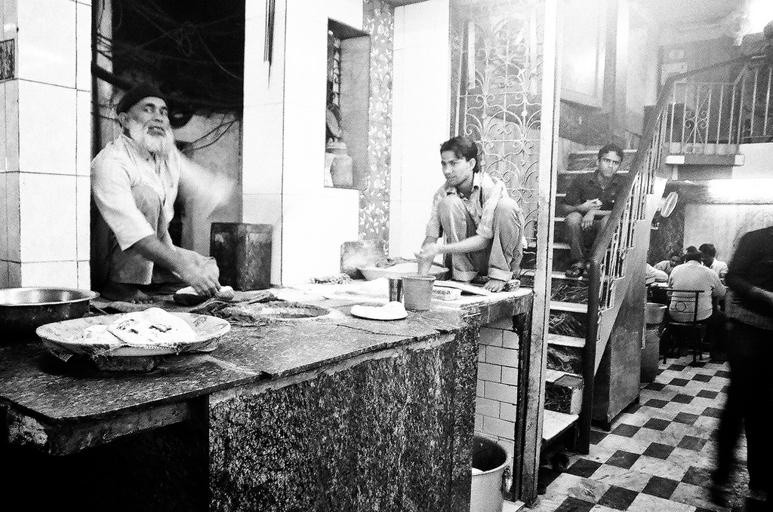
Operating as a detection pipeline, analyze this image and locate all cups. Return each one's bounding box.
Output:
[388,277,404,302]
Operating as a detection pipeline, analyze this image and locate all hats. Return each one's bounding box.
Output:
[114,78,171,119]
[683,245,703,257]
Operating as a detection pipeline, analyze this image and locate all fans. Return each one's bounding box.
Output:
[657,190,679,220]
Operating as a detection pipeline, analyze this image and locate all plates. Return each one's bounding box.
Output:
[349,305,408,321]
[34,310,231,355]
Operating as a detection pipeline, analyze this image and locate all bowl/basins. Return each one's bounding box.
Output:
[0,286,101,330]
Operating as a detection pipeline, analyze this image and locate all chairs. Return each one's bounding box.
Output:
[655,285,706,366]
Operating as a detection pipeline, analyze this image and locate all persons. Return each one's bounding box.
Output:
[413,135,525,294]
[562,142,625,280]
[653,246,684,276]
[702,223,773,497]
[91,85,221,297]
[665,245,727,363]
[697,243,729,276]
[644,260,667,285]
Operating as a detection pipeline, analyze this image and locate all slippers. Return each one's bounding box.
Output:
[565,263,583,278]
[582,262,592,277]
